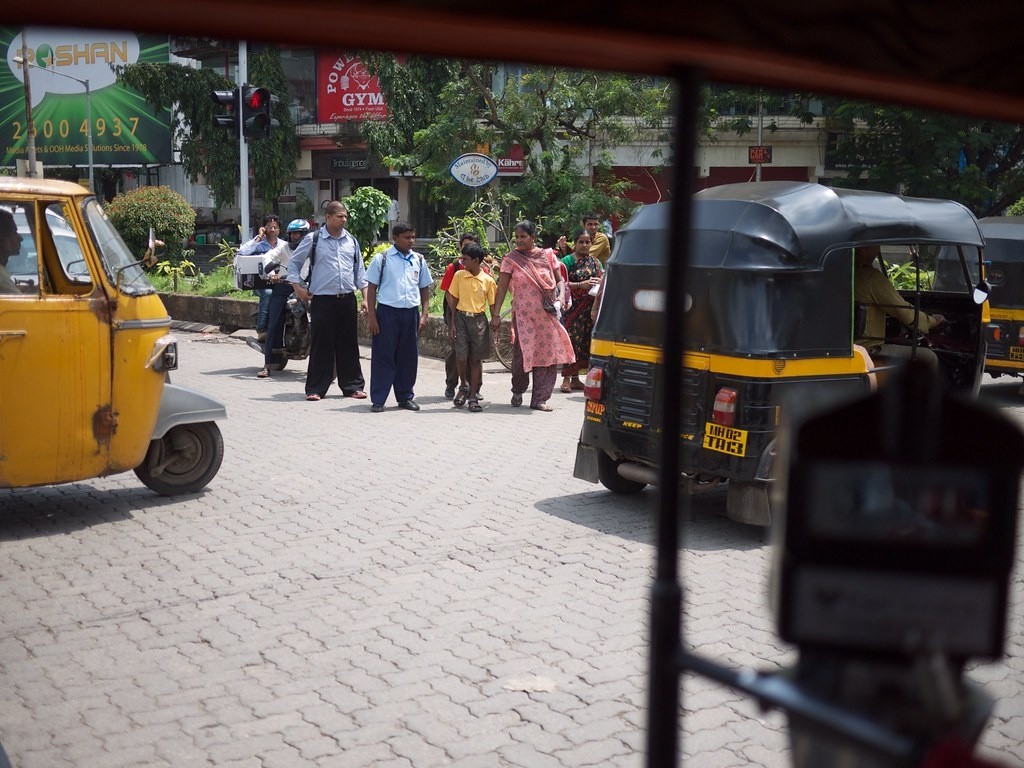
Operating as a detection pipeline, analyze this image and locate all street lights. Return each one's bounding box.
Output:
[13,57,94,194]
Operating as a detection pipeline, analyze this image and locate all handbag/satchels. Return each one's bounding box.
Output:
[543,297,562,313]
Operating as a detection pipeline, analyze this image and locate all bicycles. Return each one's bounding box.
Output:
[492,308,515,371]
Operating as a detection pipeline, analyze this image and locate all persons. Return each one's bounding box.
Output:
[440,232,488,400]
[286,201,368,401]
[490,220,576,412]
[237,213,310,378]
[0,208,24,294]
[362,220,434,413]
[855,245,945,404]
[448,241,500,411]
[557,210,613,393]
[385,193,400,240]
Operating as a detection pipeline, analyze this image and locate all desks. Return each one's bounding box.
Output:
[194,221,236,236]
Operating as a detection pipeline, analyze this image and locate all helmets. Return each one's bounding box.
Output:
[287,219,311,250]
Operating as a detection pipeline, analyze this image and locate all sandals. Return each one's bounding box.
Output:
[511,392,522,407]
[530,403,553,411]
[467,394,483,412]
[454,385,469,407]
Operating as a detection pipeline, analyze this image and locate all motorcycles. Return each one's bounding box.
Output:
[0,176,226,497]
[244,279,313,369]
[931,216,1024,381]
[571,180,990,536]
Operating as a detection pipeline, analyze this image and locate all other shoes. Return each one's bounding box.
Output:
[560,376,584,393]
[467,388,484,400]
[344,391,367,398]
[257,365,272,377]
[371,403,383,412]
[445,385,455,399]
[257,328,265,342]
[305,392,321,400]
[398,399,420,410]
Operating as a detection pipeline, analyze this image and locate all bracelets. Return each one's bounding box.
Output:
[493,313,500,317]
[591,308,598,310]
[577,282,581,289]
[560,245,567,250]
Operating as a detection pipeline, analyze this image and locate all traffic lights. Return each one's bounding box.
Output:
[212,89,239,141]
[241,85,272,141]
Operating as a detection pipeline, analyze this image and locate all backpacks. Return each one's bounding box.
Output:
[443,262,459,325]
[545,247,571,309]
